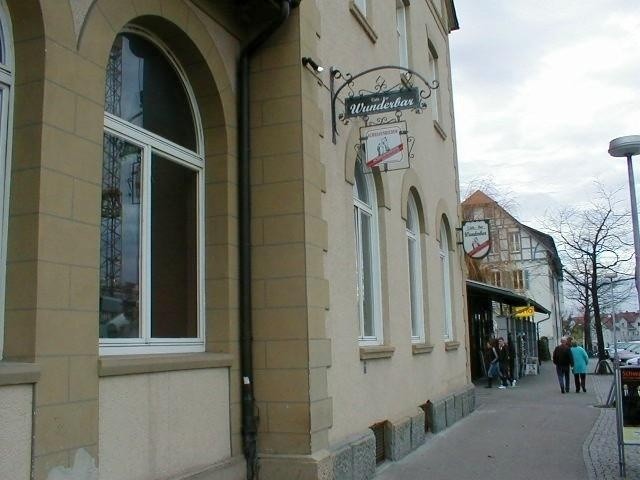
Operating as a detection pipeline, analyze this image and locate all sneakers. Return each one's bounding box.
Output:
[498,385,506,389]
[582,386,586,392]
[511,380,516,387]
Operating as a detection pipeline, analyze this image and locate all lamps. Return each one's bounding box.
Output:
[303,56,324,73]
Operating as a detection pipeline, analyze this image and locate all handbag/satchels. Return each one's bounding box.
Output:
[488,361,498,378]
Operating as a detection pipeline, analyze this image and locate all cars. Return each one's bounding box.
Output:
[604,341,640,366]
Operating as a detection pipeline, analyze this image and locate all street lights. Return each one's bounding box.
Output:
[605,272,617,353]
[608,135,640,303]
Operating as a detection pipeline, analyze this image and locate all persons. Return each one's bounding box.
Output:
[569,341,590,394]
[552,336,575,394]
[496,337,517,389]
[483,340,509,389]
